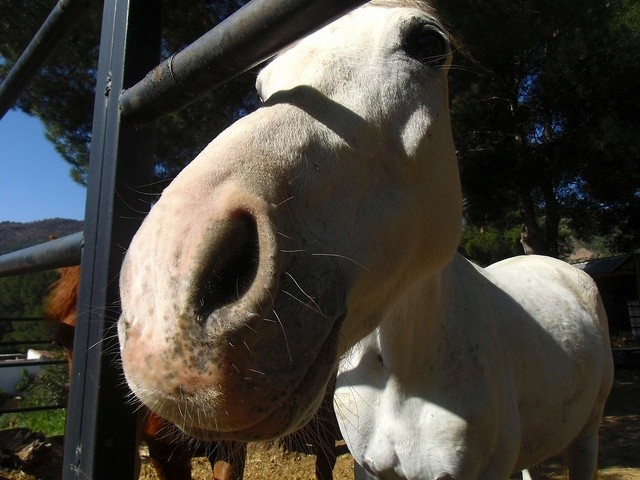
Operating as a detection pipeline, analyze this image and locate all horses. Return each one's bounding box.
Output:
[46,233,344,480]
[71,0,616,480]
